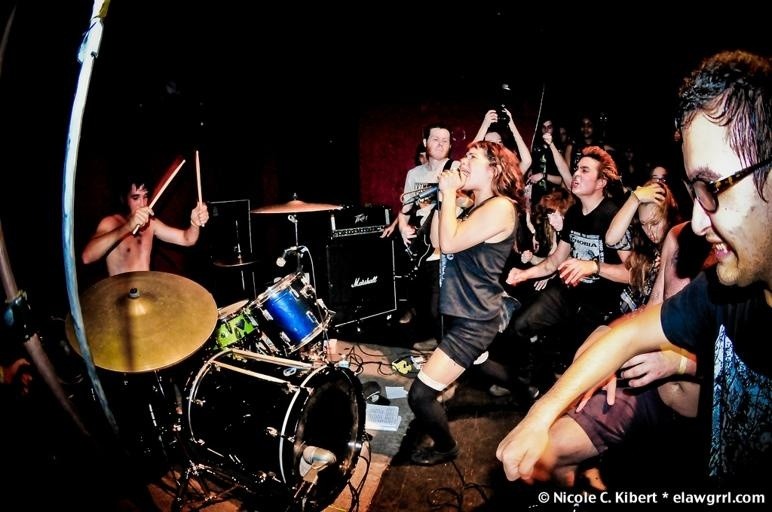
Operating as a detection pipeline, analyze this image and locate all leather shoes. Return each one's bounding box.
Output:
[413,442,459,465]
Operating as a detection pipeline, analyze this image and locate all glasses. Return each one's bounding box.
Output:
[683,158,772,213]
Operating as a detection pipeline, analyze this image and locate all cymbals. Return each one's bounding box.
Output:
[251,199,344,214]
[66,271,218,373]
[213,260,256,268]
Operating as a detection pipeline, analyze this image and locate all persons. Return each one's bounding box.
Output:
[81,167,210,276]
[379,50,769,510]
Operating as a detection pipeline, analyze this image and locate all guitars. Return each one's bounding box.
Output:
[402,197,443,281]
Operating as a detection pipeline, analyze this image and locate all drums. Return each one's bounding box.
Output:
[248,271,333,356]
[183,349,364,512]
[213,300,259,349]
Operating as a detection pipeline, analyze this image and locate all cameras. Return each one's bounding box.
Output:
[493,108,509,127]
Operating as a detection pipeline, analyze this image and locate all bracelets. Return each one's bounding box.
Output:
[189,220,199,230]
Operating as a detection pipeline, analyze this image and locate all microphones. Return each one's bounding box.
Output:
[275,251,288,268]
[405,168,460,205]
[303,446,337,465]
